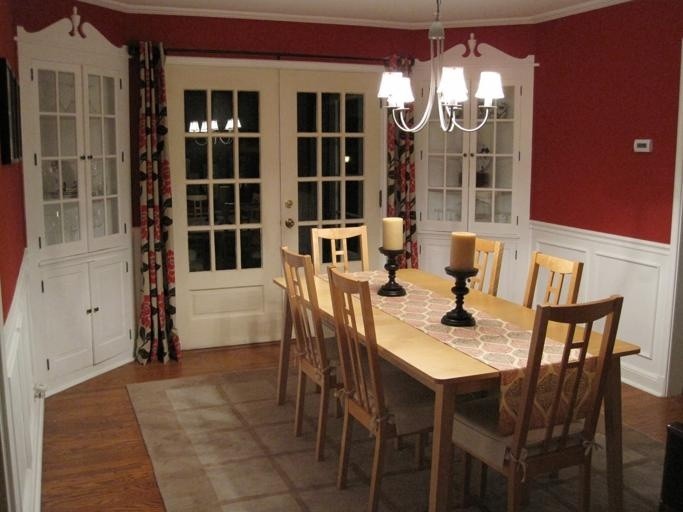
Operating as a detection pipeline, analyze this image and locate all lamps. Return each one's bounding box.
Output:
[377,1,505,134]
[188,117,241,146]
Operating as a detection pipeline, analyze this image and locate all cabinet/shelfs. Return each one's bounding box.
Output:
[414,32,536,306]
[15,6,136,397]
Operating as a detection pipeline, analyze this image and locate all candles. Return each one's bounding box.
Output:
[382,216,404,250]
[449,231,477,271]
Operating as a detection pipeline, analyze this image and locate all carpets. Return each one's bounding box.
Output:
[126,360,666,512]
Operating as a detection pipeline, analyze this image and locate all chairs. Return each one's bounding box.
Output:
[325,263,489,512]
[447,295,623,511]
[466,237,504,297]
[522,249,585,323]
[310,225,367,277]
[280,247,402,462]
